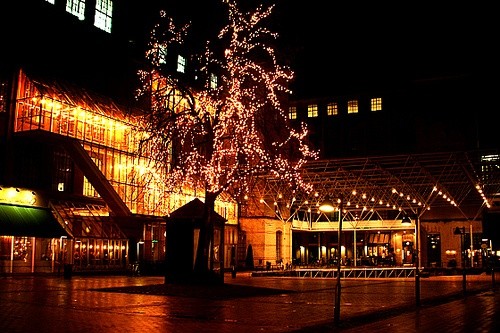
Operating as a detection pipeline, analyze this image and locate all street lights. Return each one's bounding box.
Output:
[400,214,420,307]
[319,194,342,317]
[453,226,467,296]
